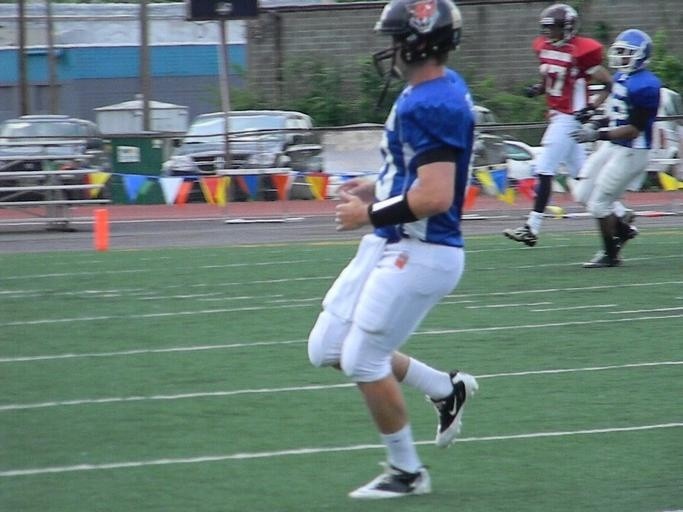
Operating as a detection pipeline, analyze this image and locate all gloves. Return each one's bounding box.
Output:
[522,84,545,97]
[566,106,602,143]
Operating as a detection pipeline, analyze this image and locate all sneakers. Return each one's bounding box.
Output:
[501,223,537,247]
[583,210,637,267]
[348,460,432,498]
[429,369,478,449]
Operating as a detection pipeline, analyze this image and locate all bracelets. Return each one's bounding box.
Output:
[369,193,419,229]
[599,127,608,140]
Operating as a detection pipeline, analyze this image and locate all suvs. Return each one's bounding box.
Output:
[164,107,325,201]
[0,114,108,202]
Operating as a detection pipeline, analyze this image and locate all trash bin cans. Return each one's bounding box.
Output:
[111,131,165,204]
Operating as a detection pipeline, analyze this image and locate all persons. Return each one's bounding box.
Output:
[502,1,639,246]
[567,29,660,268]
[308,0,475,499]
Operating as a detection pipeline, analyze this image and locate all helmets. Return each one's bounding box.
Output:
[373,0,462,65]
[540,4,580,47]
[606,28,653,73]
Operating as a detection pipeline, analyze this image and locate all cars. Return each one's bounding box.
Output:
[640,88,683,189]
[468,108,549,187]
